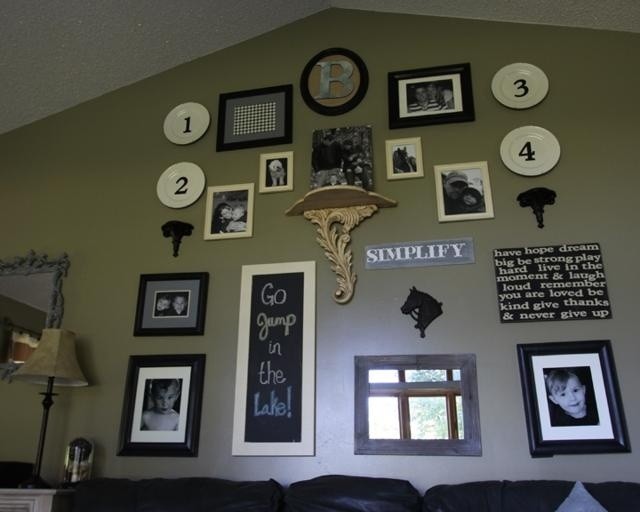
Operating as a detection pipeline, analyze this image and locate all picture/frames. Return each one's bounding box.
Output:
[133,272,208,335]
[385,137,426,180]
[515,340,632,456]
[203,184,253,241]
[259,149,293,192]
[216,84,293,151]
[115,354,206,457]
[434,161,495,222]
[387,63,475,129]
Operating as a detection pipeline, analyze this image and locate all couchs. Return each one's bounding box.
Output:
[71,475,640,512]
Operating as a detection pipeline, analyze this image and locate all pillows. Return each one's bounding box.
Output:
[556,481,609,512]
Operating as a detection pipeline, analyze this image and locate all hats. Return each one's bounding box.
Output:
[467,177,483,196]
[445,170,469,184]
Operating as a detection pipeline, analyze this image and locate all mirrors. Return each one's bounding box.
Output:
[0,250,69,364]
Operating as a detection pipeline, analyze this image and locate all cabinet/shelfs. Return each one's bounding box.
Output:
[0,488,73,512]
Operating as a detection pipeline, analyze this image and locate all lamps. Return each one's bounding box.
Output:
[9,328,90,490]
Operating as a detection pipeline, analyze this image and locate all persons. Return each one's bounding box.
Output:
[427,84,447,110]
[408,87,441,111]
[463,186,482,206]
[142,378,180,432]
[546,369,599,426]
[313,134,342,185]
[213,204,233,233]
[171,293,188,315]
[154,295,171,316]
[443,170,468,215]
[339,139,373,190]
[227,204,246,233]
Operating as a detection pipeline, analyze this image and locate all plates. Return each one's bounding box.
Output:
[156,162,205,209]
[499,124,562,177]
[163,102,210,145]
[490,61,550,111]
